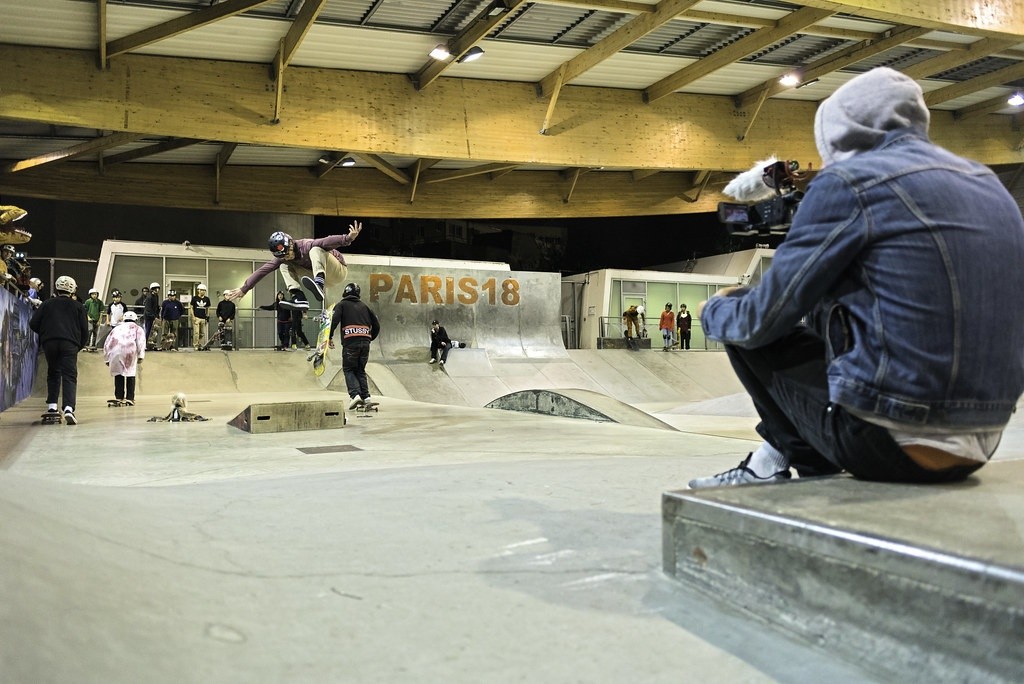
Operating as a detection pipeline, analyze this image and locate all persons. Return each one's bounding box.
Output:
[190,284,211,351]
[623,305,647,339]
[29,275,88,425]
[0,205,44,311]
[144,282,162,351]
[104,311,146,406]
[216,290,235,346]
[85,288,104,350]
[257,291,290,348]
[688,66,1023,490]
[659,302,675,349]
[71,293,83,303]
[291,310,310,352]
[228,220,362,310]
[133,287,149,324]
[429,320,452,366]
[329,282,380,409]
[105,291,128,329]
[677,304,692,350]
[160,289,184,351]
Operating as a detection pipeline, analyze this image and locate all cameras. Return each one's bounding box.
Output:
[716,159,811,239]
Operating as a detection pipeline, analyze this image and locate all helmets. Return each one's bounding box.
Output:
[28,277,43,291]
[150,282,160,289]
[636,306,644,315]
[123,311,138,323]
[267,230,293,257]
[197,284,206,290]
[167,289,177,297]
[54,276,77,293]
[88,287,100,295]
[111,291,122,299]
[222,290,233,295]
[342,282,361,300]
[2,244,15,253]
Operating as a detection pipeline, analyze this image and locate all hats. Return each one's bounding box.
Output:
[431,319,439,325]
[277,290,284,295]
[666,303,672,308]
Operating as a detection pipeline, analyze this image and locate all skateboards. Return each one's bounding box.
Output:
[108,399,127,406]
[357,402,379,413]
[199,332,220,350]
[83,346,98,352]
[41,411,61,424]
[306,302,337,376]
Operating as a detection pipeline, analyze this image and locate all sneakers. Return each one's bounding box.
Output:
[301,275,325,302]
[64,410,79,426]
[364,396,372,405]
[48,406,61,417]
[349,394,363,411]
[278,295,310,311]
[430,357,444,366]
[687,452,793,494]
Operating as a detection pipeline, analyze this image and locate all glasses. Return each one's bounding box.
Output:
[433,323,437,326]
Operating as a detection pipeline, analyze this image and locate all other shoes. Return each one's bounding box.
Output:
[116,396,134,405]
[292,344,311,350]
